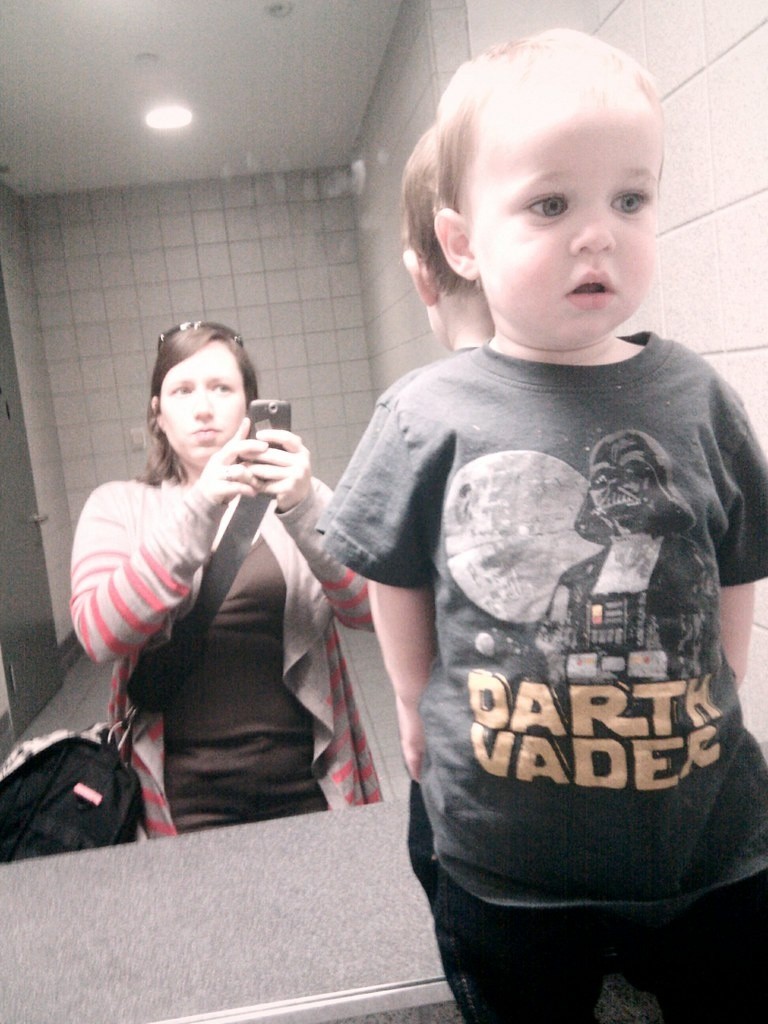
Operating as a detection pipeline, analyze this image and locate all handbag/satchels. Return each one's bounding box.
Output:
[1,722,143,864]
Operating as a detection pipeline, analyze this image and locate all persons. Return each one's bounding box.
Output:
[67,321,385,844]
[312,121,494,912]
[317,28,768,1024]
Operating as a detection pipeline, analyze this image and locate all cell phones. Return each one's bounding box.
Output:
[244,399,293,481]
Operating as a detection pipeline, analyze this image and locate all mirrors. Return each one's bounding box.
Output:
[0,0,768,1024]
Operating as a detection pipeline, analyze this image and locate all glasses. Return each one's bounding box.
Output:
[158,321,243,349]
